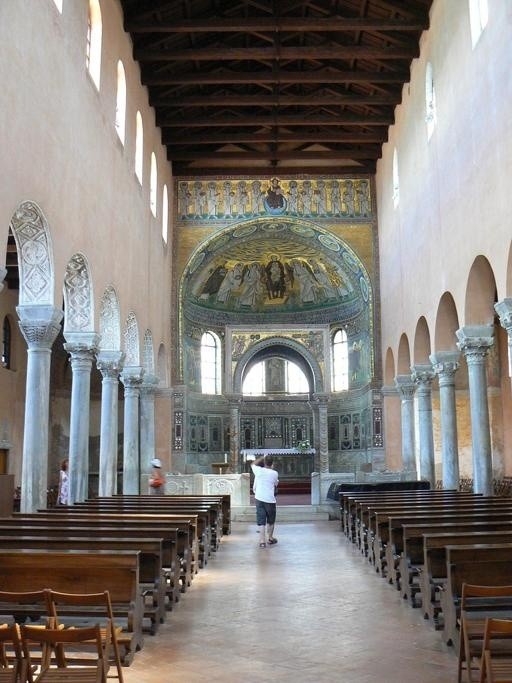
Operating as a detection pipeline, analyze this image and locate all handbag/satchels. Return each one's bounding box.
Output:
[148,479,160,487]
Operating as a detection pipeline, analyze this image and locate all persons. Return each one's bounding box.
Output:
[148,459,166,495]
[250,455,279,547]
[178,178,369,220]
[57,459,68,505]
[201,254,350,308]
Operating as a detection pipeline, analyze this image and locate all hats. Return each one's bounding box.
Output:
[150,459,161,468]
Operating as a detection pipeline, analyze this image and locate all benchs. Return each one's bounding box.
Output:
[337,487,512,648]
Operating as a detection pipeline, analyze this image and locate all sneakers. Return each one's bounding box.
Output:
[259,543,265,548]
[267,539,277,544]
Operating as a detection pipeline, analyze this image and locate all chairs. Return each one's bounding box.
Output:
[477,616,511,682]
[456,583,511,683]
[0,622,38,683]
[0,589,64,666]
[47,588,124,683]
[18,623,111,683]
